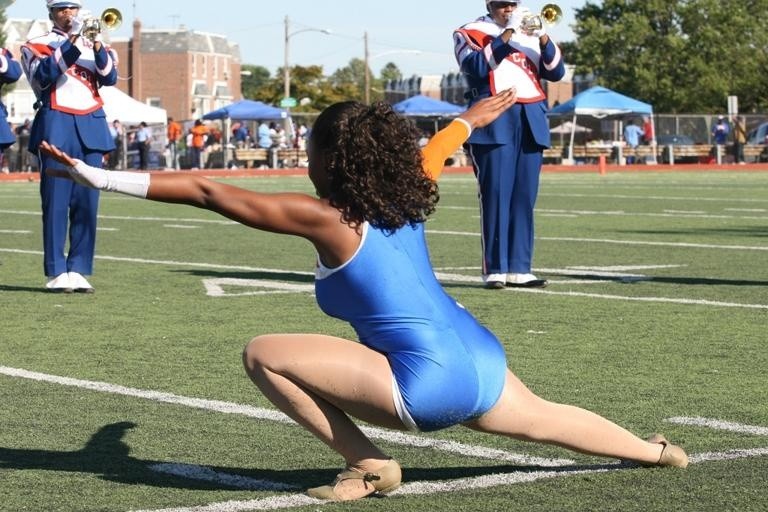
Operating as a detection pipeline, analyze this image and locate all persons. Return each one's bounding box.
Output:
[451,0,566,289]
[623,108,749,165]
[19,0,121,295]
[40,84,689,503]
[1,118,309,172]
[0,46,22,171]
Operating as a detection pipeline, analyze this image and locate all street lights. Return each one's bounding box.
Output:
[364,48,420,110]
[286,28,333,99]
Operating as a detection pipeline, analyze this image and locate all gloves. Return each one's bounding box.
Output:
[71,9,101,43]
[505,7,546,38]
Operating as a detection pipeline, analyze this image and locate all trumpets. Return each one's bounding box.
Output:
[506,4,563,35]
[68,8,123,43]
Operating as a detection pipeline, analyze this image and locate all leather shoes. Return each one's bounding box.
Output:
[74,288,95,293]
[506,280,548,288]
[487,281,503,289]
[308,459,402,502]
[645,434,689,469]
[49,288,72,292]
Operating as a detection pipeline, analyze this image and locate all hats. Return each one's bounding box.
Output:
[46,1,81,7]
